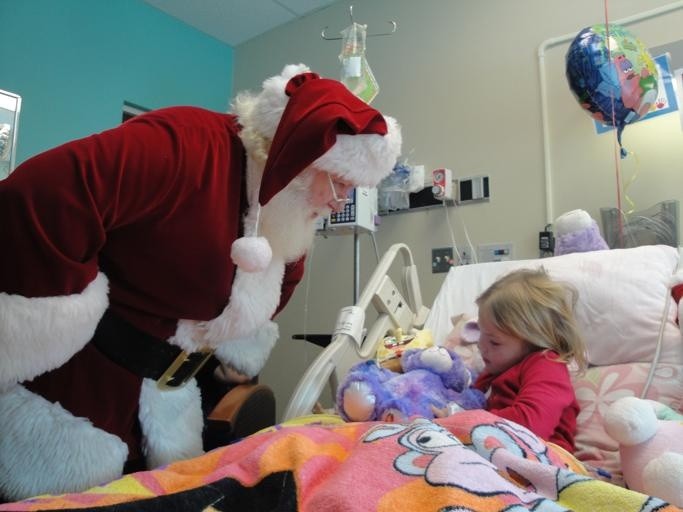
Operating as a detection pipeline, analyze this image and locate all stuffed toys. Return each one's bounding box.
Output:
[606,397,683,509]
[554,210,610,256]
[336,345,488,422]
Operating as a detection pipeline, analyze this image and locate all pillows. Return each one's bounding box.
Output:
[429,247,683,362]
[553,364,683,490]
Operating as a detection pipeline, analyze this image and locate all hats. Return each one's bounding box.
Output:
[229,64,402,272]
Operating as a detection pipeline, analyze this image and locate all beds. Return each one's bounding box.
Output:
[1,242,683,512]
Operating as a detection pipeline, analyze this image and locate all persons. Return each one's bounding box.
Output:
[0,65,400,501]
[433,269,580,454]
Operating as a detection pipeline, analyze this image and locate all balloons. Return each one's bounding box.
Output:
[566,24,658,126]
